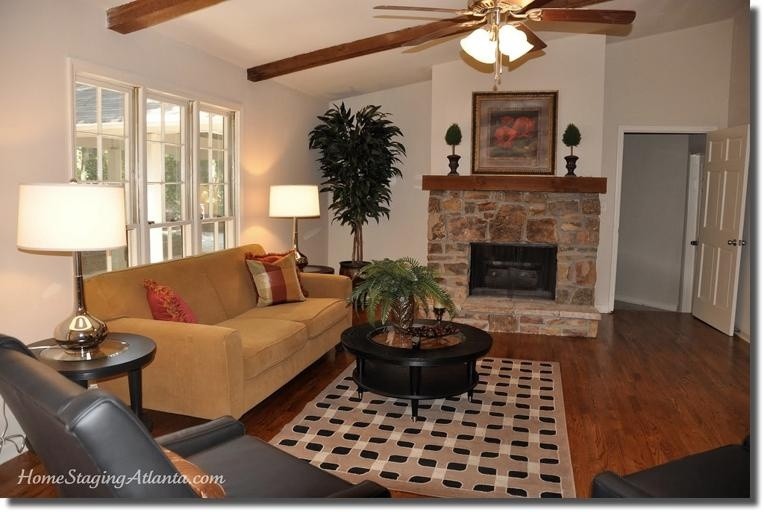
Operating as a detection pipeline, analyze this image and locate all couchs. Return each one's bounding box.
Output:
[80,239,358,420]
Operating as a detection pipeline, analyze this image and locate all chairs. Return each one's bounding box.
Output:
[1,332,393,501]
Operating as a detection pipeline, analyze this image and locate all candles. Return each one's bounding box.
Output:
[432,284,445,308]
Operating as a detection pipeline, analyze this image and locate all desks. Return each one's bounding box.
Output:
[297,264,333,275]
[24,327,157,432]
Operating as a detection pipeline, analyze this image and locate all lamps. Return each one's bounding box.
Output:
[459,11,536,87]
[14,178,130,353]
[266,183,322,269]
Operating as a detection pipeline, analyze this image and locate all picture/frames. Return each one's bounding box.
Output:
[470,90,560,174]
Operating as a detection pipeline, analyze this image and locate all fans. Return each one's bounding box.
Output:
[372,0,638,53]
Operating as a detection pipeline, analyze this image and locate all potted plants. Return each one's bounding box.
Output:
[342,255,462,337]
[443,122,463,177]
[306,98,406,308]
[559,122,583,178]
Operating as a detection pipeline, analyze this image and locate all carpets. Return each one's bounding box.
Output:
[263,348,580,498]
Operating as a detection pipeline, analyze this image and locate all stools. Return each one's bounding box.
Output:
[586,429,750,498]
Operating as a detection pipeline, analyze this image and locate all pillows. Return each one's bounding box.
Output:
[141,278,198,324]
[243,247,309,305]
[243,251,306,309]
[155,442,230,499]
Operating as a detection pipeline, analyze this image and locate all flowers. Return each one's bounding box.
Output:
[489,111,536,158]
